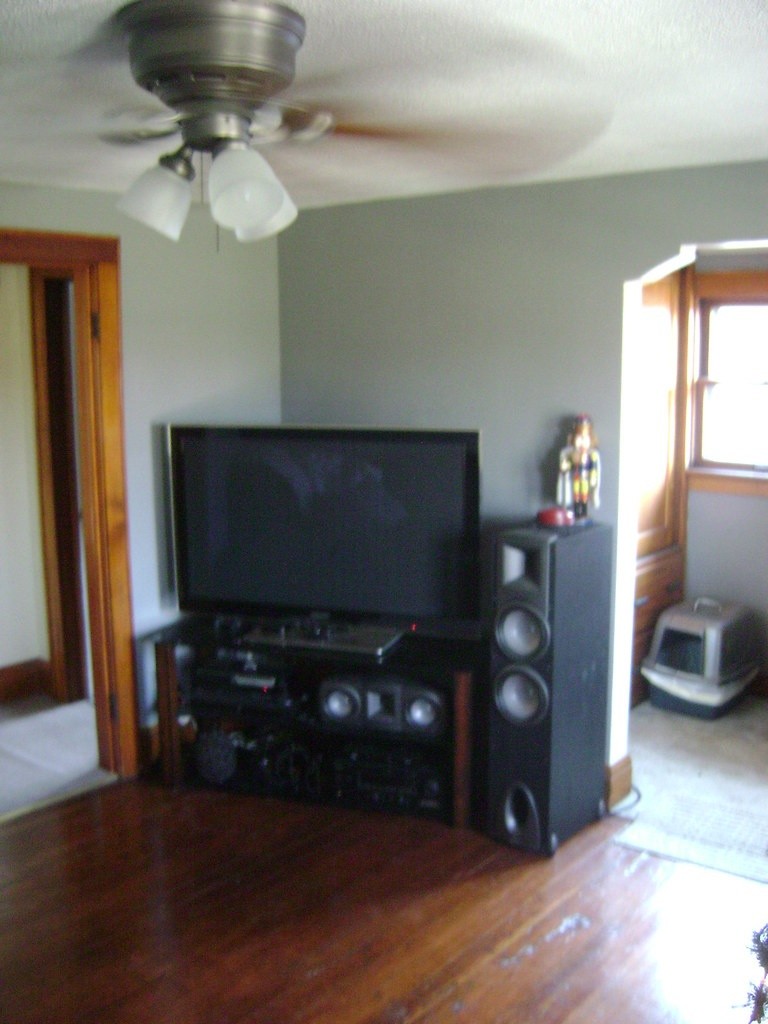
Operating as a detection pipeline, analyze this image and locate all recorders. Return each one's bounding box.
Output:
[318,675,447,736]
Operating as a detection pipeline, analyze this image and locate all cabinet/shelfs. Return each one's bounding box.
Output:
[629,260,693,708]
[140,613,485,830]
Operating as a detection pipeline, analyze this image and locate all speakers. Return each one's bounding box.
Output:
[487,523,610,856]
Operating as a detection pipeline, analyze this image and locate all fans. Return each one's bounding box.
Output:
[2,0,613,193]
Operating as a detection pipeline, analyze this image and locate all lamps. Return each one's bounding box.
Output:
[116,110,298,254]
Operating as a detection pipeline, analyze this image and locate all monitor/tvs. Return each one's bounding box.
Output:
[166,424,479,655]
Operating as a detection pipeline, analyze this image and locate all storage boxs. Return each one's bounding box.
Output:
[640,595,758,721]
